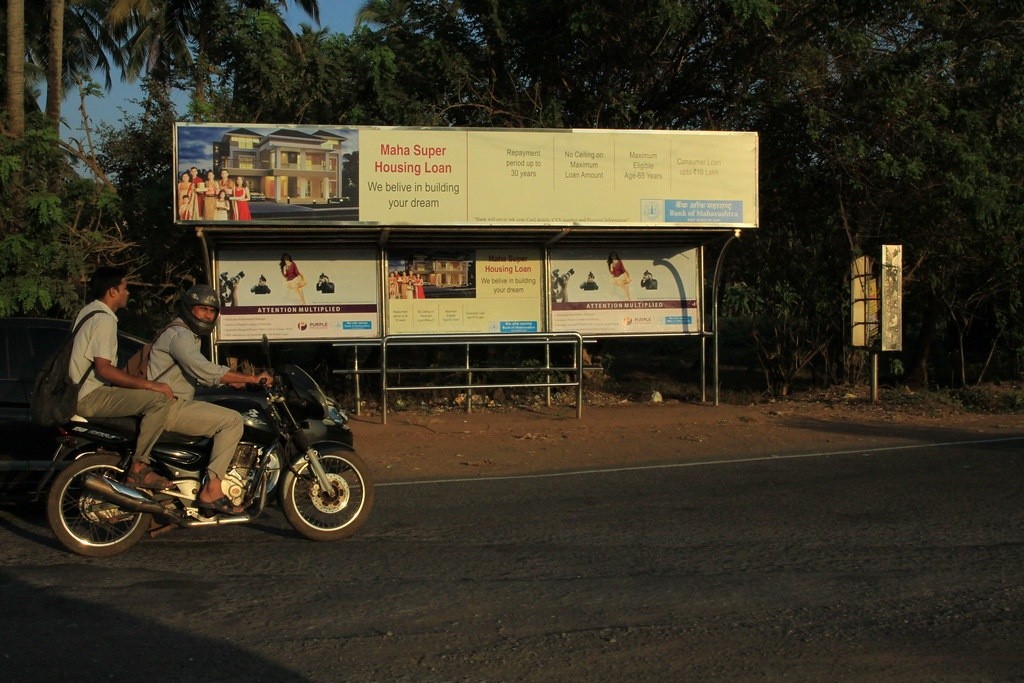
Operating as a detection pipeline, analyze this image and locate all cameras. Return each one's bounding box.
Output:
[552,268,574,286]
[220,271,245,286]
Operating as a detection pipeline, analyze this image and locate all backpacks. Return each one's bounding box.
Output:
[123,322,190,383]
[27,309,108,427]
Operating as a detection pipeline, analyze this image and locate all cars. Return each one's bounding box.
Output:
[1,314,356,517]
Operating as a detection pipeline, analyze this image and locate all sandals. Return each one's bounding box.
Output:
[124,465,176,489]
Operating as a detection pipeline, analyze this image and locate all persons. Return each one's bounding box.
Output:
[553,252,658,304]
[147,284,272,515]
[390,271,422,300]
[221,254,334,306]
[65,266,177,490]
[179,167,252,220]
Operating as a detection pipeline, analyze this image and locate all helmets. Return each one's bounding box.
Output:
[177,285,220,336]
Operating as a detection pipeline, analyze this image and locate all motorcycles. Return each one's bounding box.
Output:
[34,332,377,561]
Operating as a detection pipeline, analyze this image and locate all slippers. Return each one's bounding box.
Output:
[191,494,244,516]
